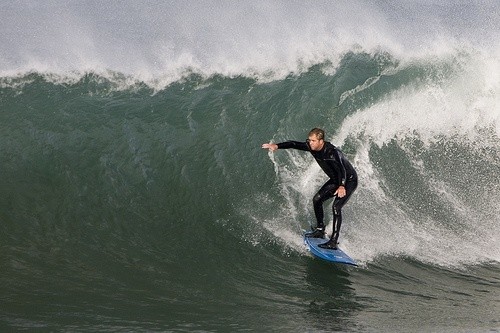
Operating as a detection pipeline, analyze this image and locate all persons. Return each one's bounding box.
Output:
[261,128,358,251]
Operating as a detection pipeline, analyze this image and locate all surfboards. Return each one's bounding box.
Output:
[304,230,358,267]
